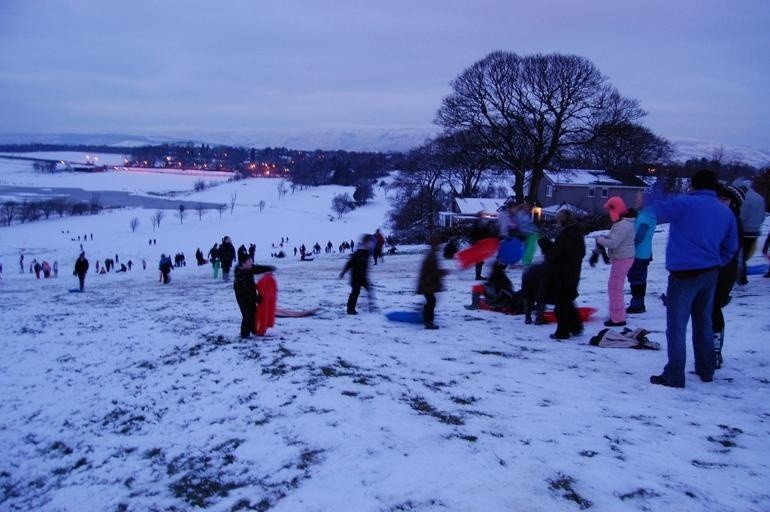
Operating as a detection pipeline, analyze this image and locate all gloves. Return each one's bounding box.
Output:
[589,243,610,267]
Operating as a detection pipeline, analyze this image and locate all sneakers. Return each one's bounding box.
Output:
[549,334,570,339]
[604,318,626,326]
[424,322,439,329]
[626,306,645,314]
[347,309,358,314]
[650,375,685,388]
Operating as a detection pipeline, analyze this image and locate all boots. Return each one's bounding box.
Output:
[464,292,479,310]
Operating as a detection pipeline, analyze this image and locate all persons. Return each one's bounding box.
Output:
[149,236,277,339]
[20,229,147,292]
[271,228,399,315]
[414,162,769,388]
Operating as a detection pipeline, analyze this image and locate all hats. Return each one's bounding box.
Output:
[720,180,752,207]
[604,196,628,222]
[691,169,719,191]
